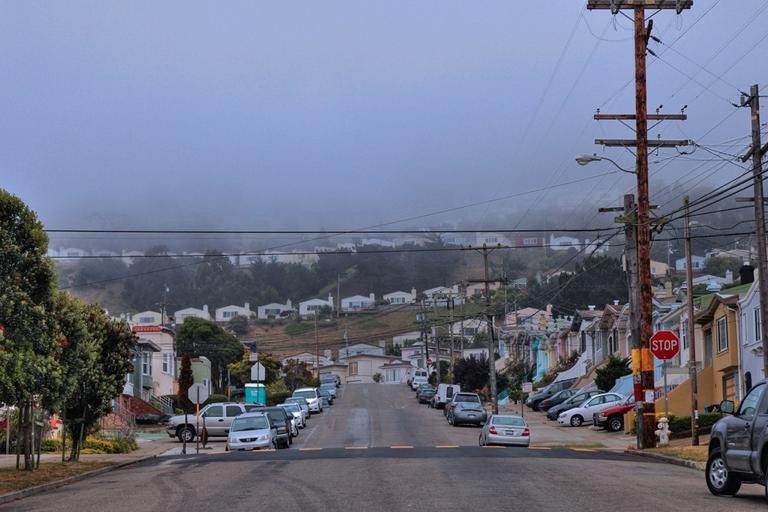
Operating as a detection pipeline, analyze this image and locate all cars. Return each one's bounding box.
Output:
[478,413,530,447]
[248,372,341,447]
[526,377,606,419]
[706,379,768,503]
[557,392,627,427]
[408,366,487,427]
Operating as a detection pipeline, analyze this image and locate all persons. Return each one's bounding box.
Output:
[49,415,62,441]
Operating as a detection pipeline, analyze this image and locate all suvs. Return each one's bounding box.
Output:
[224,411,278,453]
[166,402,271,441]
[592,388,664,433]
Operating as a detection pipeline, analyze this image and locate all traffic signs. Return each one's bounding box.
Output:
[649,330,682,362]
